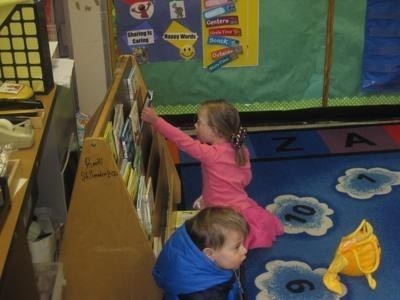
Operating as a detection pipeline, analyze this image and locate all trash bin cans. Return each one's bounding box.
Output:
[26,232,57,293]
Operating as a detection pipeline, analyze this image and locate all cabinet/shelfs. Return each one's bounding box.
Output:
[60,51,183,299]
[0,0,83,299]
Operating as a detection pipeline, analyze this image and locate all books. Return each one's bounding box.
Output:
[102,62,163,258]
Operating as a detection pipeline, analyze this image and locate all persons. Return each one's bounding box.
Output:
[152,206,250,300]
[140,98,284,251]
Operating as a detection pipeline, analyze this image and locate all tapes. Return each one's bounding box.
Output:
[0,119,26,131]
